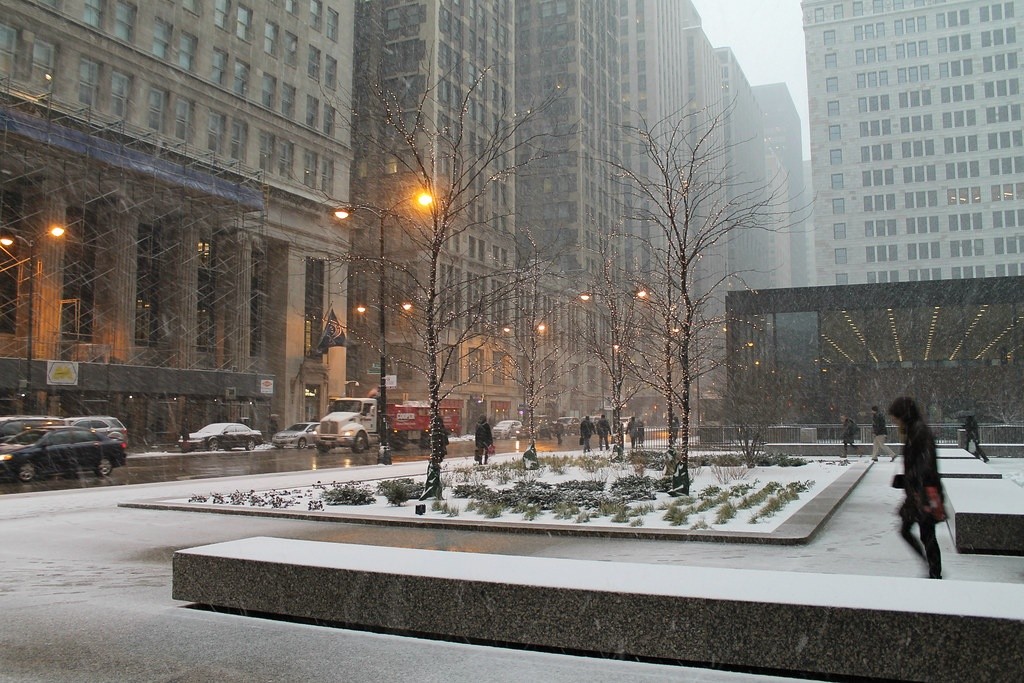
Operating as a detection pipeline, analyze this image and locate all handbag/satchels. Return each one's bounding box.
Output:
[474,449,481,462]
[968,440,976,454]
[848,419,858,435]
[580,437,584,446]
[488,445,495,458]
[914,486,947,524]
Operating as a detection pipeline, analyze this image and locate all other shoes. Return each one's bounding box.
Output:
[890,454,898,463]
[984,458,989,463]
[840,455,846,458]
[872,458,879,462]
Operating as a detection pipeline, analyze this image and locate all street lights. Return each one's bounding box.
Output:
[0,223,66,416]
[580,290,647,415]
[357,302,412,433]
[334,192,432,461]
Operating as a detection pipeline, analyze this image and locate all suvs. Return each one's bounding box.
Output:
[555,417,581,436]
[523,415,552,438]
[579,416,635,432]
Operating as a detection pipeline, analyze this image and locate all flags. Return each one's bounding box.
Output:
[316,311,361,358]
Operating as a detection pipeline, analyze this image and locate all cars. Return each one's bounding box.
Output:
[0,415,128,449]
[177,423,264,454]
[271,422,322,448]
[492,420,523,440]
[0,426,129,483]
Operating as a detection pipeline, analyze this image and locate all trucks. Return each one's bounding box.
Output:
[313,397,461,454]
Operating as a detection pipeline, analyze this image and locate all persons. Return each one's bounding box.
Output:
[624,415,645,448]
[837,414,864,458]
[869,404,898,463]
[554,419,564,445]
[961,415,990,464]
[474,415,494,465]
[887,395,943,579]
[596,415,610,451]
[579,415,596,452]
[671,411,680,445]
